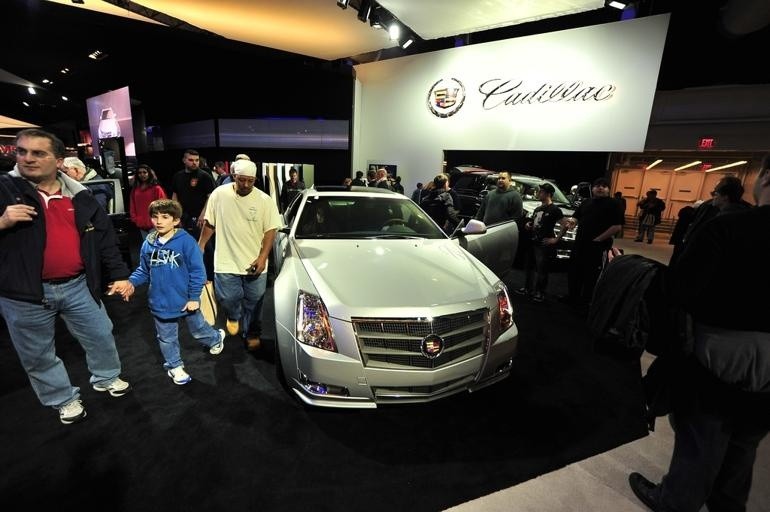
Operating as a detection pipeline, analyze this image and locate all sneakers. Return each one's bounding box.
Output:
[514,287,534,295]
[209,328,226,355]
[58,398,87,425]
[246,335,261,350]
[168,365,192,385]
[92,376,132,397]
[532,291,544,302]
[226,317,239,335]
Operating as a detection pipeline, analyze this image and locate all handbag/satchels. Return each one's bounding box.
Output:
[643,213,656,226]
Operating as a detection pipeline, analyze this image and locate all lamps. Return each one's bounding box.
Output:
[22,50,107,107]
[605,1,629,11]
[337,0,415,49]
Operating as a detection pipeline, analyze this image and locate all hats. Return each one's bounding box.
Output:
[234,159,257,177]
[539,183,555,192]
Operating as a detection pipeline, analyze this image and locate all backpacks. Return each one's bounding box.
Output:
[420,192,448,228]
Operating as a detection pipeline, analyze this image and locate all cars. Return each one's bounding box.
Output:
[452,166,578,263]
[268,184,521,406]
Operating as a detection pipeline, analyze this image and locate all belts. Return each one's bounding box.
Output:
[42,272,82,285]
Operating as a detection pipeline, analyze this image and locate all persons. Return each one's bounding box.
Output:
[475,172,522,226]
[93,107,123,144]
[0,127,131,426]
[602,150,770,512]
[514,183,568,301]
[1,132,305,270]
[195,158,280,349]
[345,168,404,218]
[112,197,231,389]
[557,178,626,306]
[412,172,461,233]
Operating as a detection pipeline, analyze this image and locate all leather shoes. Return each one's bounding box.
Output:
[629,473,671,512]
